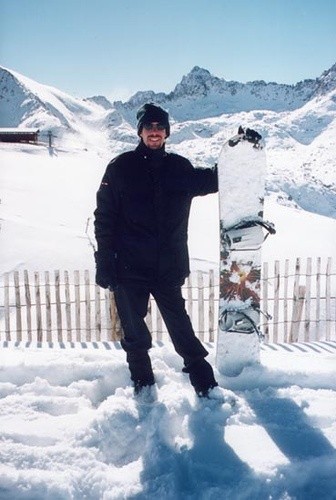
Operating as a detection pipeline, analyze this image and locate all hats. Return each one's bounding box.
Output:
[136,104,170,138]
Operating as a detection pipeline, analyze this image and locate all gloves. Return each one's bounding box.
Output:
[238,125,262,144]
[94,249,119,288]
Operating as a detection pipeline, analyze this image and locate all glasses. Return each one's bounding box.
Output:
[144,122,165,130]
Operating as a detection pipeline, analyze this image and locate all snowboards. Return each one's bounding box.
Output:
[216,134,276,377]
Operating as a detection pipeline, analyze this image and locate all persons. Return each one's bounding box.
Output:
[94,102,262,398]
